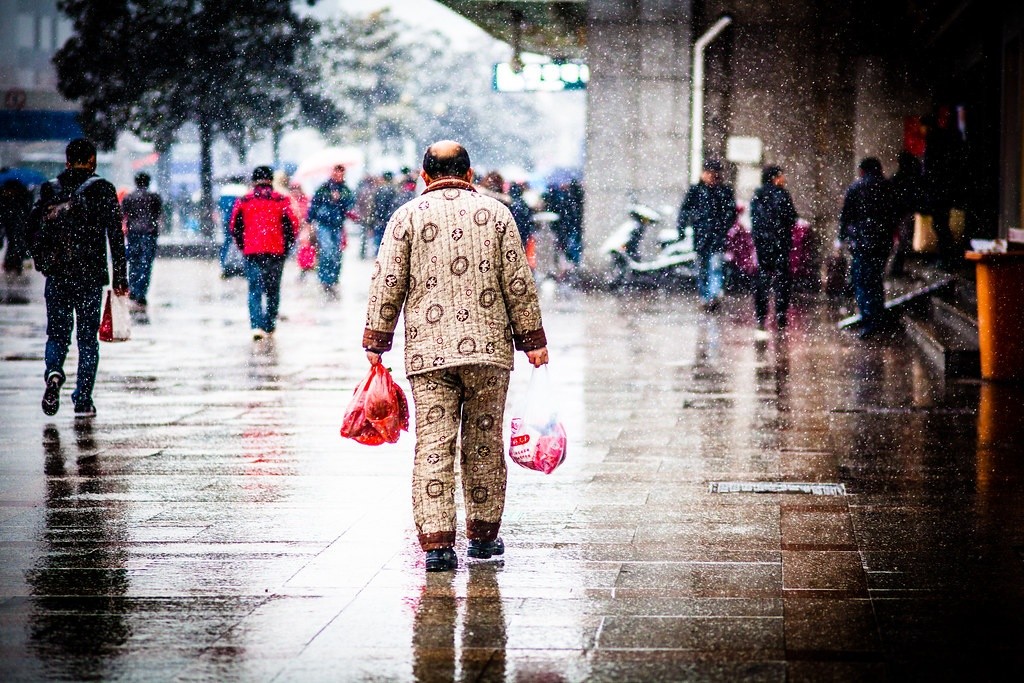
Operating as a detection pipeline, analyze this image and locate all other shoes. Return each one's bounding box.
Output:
[255,327,274,340]
[42,372,62,416]
[74,402,96,418]
[424,550,457,572]
[466,535,505,558]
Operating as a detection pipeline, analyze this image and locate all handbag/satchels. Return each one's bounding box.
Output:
[340,363,410,446]
[98,292,132,344]
[509,366,568,475]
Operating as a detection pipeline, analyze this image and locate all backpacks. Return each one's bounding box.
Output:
[32,174,106,280]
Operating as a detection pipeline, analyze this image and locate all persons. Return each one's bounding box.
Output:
[120,172,163,325]
[839,158,889,340]
[676,160,738,314]
[0,166,34,286]
[751,167,799,340]
[304,163,355,297]
[40,138,129,419]
[356,166,583,269]
[218,166,307,342]
[362,140,549,570]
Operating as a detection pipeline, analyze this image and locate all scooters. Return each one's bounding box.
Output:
[600,204,699,289]
